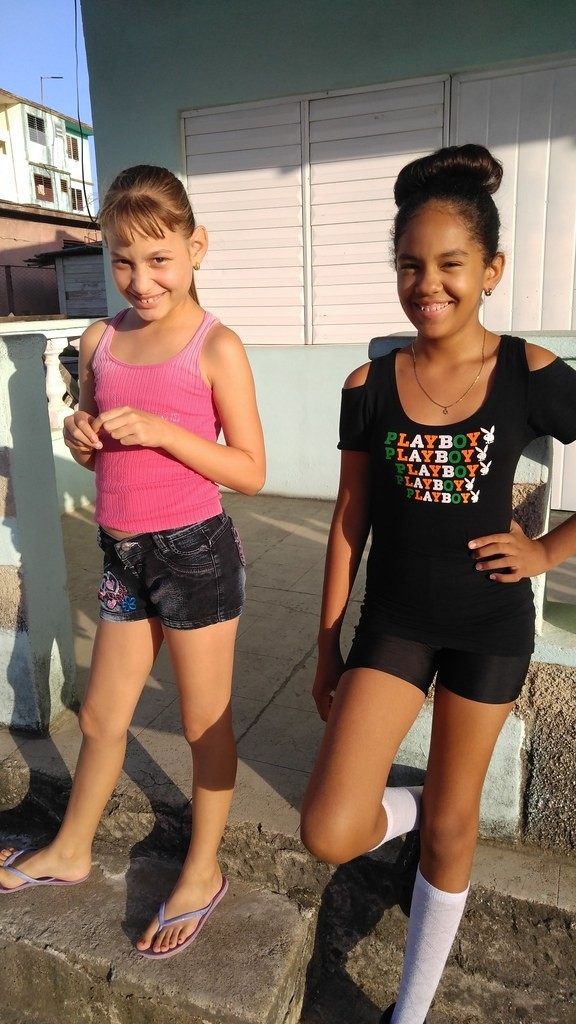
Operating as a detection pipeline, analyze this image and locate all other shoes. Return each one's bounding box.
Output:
[378,1002,427,1024]
[391,831,422,917]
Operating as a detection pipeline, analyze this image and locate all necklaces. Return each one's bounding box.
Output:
[411,329,486,415]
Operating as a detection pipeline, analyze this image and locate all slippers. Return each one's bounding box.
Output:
[136,875,230,959]
[0,847,92,893]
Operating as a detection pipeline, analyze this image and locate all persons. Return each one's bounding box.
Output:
[0,165,266,960]
[300,142,576,1024]
